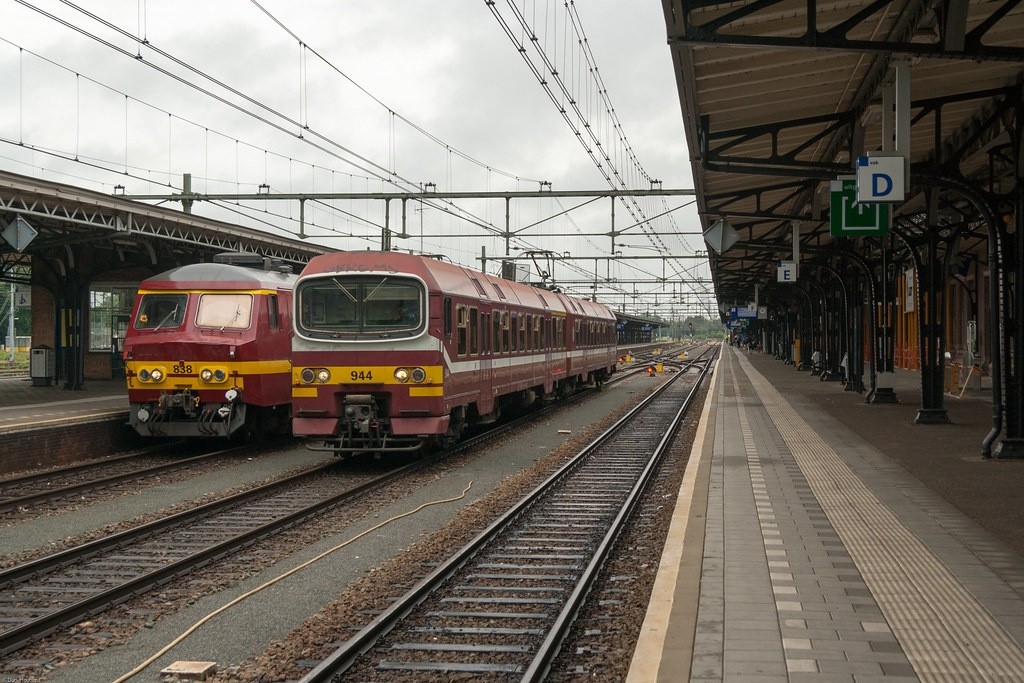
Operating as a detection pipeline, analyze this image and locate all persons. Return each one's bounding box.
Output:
[732,334,761,353]
[810,348,822,369]
[839,352,848,384]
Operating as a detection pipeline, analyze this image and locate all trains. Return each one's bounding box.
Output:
[290,249,619,460]
[121,249,326,445]
